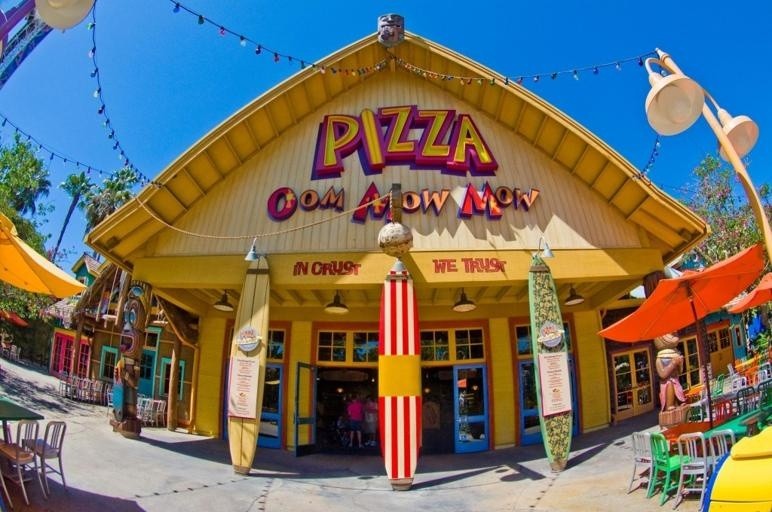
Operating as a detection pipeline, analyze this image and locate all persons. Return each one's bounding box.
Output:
[335,393,378,448]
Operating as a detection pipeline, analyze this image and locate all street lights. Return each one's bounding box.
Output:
[643,45,771,267]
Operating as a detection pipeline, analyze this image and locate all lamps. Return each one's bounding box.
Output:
[243,236,261,263]
[452,286,477,312]
[212,289,233,313]
[565,285,584,306]
[534,235,553,261]
[323,290,349,317]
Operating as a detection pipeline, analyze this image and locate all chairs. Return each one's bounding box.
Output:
[37,421,66,493]
[107,391,166,428]
[0,342,23,363]
[59,371,109,404]
[1,421,49,505]
[630,345,771,512]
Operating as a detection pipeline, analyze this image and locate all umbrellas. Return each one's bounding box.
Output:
[729,272,772,313]
[599,243,767,425]
[0,211,89,302]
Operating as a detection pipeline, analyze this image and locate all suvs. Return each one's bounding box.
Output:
[466,375,484,440]
